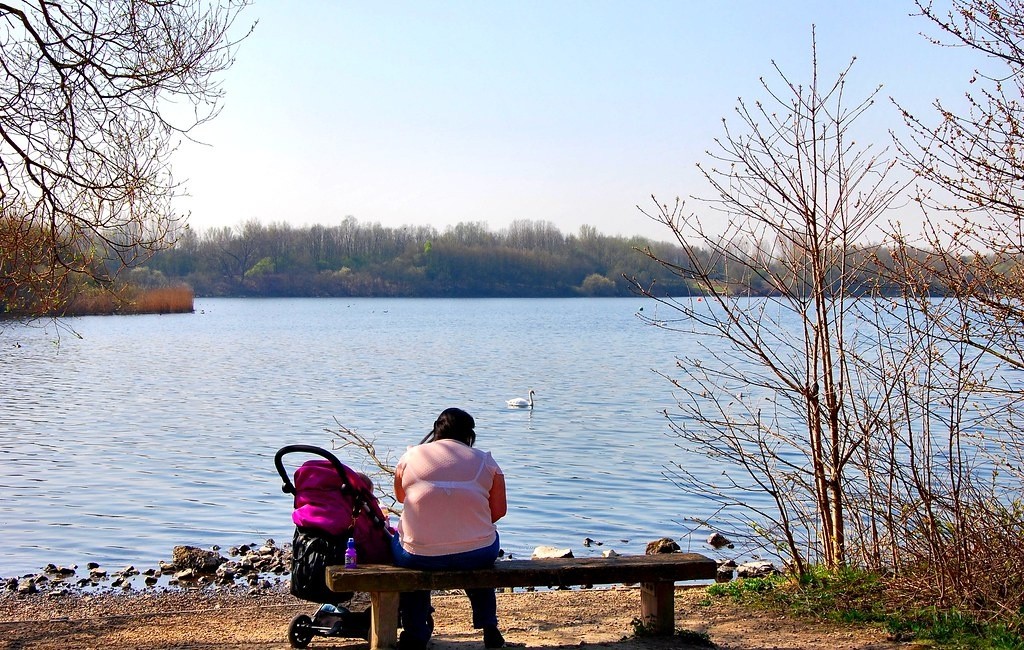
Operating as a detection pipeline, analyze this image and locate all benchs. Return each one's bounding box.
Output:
[325,552,717,650]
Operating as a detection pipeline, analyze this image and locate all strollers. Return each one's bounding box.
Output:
[273,445,436,648]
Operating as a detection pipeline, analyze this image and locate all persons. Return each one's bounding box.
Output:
[392,408,507,648]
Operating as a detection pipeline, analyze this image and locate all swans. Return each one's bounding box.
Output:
[505,389,536,406]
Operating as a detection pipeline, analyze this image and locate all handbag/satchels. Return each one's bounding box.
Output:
[289,524,356,604]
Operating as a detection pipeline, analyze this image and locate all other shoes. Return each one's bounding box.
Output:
[483,626,504,648]
[400,632,427,650]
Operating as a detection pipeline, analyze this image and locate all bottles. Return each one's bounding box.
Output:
[345,538,357,568]
